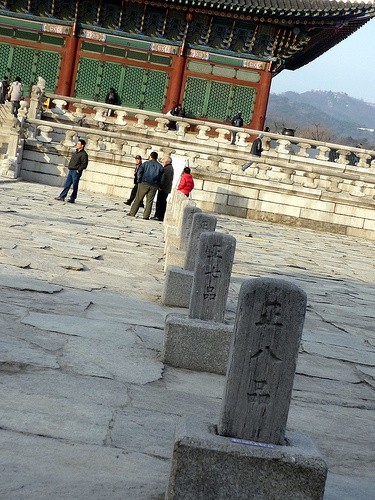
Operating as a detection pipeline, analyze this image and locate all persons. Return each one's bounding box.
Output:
[8,76,23,118]
[55,139,88,203]
[0,76,10,104]
[104,88,118,116]
[126,152,164,220]
[177,167,194,197]
[124,155,145,208]
[231,111,243,145]
[166,104,182,130]
[149,157,174,221]
[264,127,271,132]
[242,134,264,174]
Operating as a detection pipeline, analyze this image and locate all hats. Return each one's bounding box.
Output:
[134,155,142,159]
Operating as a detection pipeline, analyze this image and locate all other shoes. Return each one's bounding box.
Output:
[126,213,135,217]
[142,217,150,220]
[123,202,131,206]
[150,216,163,221]
[67,199,74,203]
[54,196,65,201]
